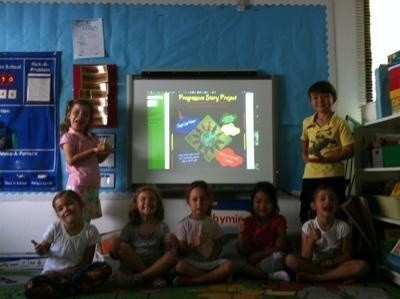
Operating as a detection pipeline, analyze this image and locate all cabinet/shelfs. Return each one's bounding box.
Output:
[353,114,400,286]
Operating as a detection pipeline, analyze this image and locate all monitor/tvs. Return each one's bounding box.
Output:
[125,68,281,193]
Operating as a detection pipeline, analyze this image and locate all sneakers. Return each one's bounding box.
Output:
[152,277,169,288]
[116,272,144,287]
[269,270,292,282]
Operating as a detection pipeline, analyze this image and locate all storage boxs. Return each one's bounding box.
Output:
[383,144,400,167]
[361,102,376,121]
[373,195,400,218]
[387,64,400,90]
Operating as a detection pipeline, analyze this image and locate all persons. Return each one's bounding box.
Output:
[283,184,375,284]
[172,180,237,286]
[108,185,178,291]
[26,189,112,298]
[224,181,288,280]
[59,98,111,223]
[299,83,355,225]
